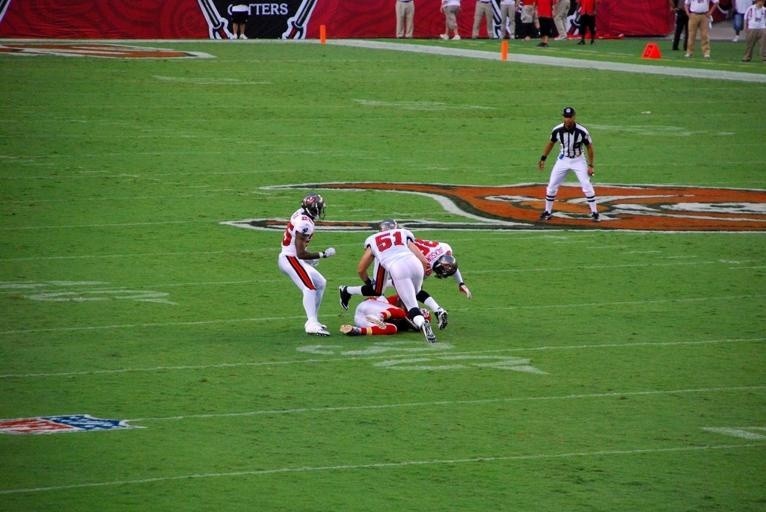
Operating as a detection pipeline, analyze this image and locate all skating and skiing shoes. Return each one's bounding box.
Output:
[421,320,436,343]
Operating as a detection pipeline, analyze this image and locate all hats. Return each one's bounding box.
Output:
[562,107,576,117]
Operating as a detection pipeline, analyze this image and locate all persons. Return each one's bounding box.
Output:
[339,238,471,312]
[471,0,599,46]
[340,295,431,336]
[670,1,766,62]
[278,194,336,336]
[395,0,415,38]
[230,0,250,40]
[358,219,447,344]
[440,0,461,40]
[538,106,601,222]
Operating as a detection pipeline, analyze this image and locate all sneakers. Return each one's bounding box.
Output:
[540,213,553,220]
[367,315,386,330]
[592,212,600,222]
[305,319,331,337]
[339,323,353,334]
[435,307,448,330]
[439,32,461,41]
[339,285,351,310]
[683,52,712,58]
[536,42,549,49]
[555,34,595,45]
[733,36,750,63]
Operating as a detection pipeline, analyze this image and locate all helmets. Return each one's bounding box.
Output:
[377,220,400,232]
[433,256,458,278]
[301,192,325,220]
[419,307,432,324]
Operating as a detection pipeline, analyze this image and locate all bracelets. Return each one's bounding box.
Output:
[540,155,546,161]
[589,164,593,168]
[318,252,324,259]
[364,278,371,285]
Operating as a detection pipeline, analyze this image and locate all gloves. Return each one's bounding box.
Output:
[459,283,472,299]
[364,278,376,285]
[319,247,336,258]
[305,259,320,267]
